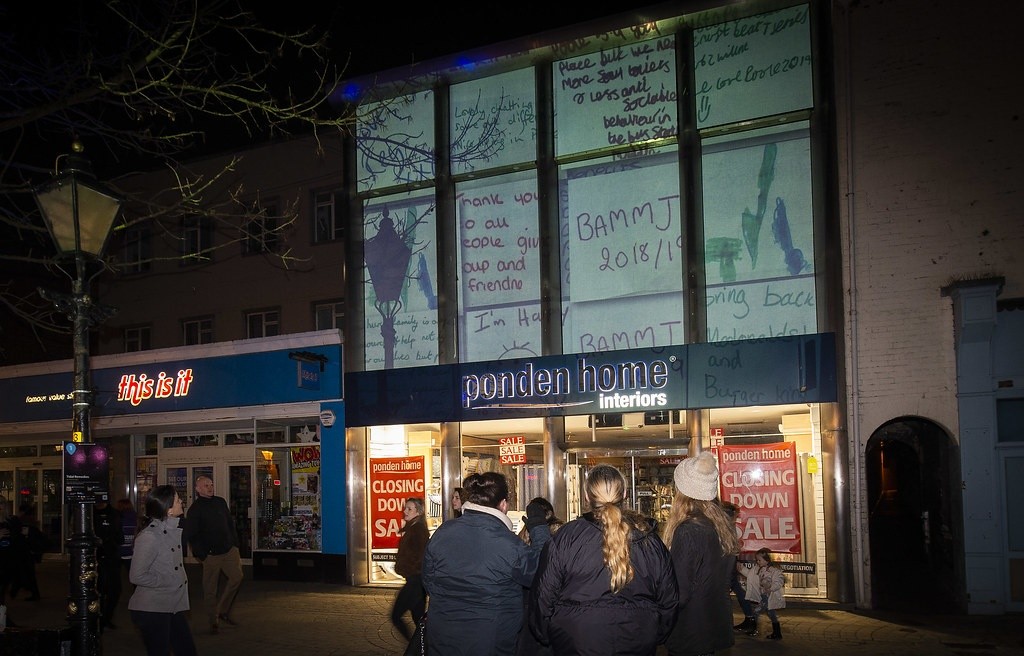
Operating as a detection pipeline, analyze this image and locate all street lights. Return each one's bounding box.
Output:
[18,140,130,656]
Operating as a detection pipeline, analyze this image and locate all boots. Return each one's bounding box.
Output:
[767,623,782,640]
[744,617,757,635]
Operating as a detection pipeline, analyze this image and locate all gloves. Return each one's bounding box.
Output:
[522,503,547,533]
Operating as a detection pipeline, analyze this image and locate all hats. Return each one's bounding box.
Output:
[674,451,719,501]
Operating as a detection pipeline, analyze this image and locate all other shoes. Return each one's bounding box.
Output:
[209,623,219,635]
[24,595,40,601]
[104,621,118,630]
[733,619,747,632]
[219,614,237,625]
[6,616,17,628]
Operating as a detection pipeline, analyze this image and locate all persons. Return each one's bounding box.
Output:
[390,453,786,656]
[186,475,244,633]
[0,493,48,627]
[93,485,199,656]
